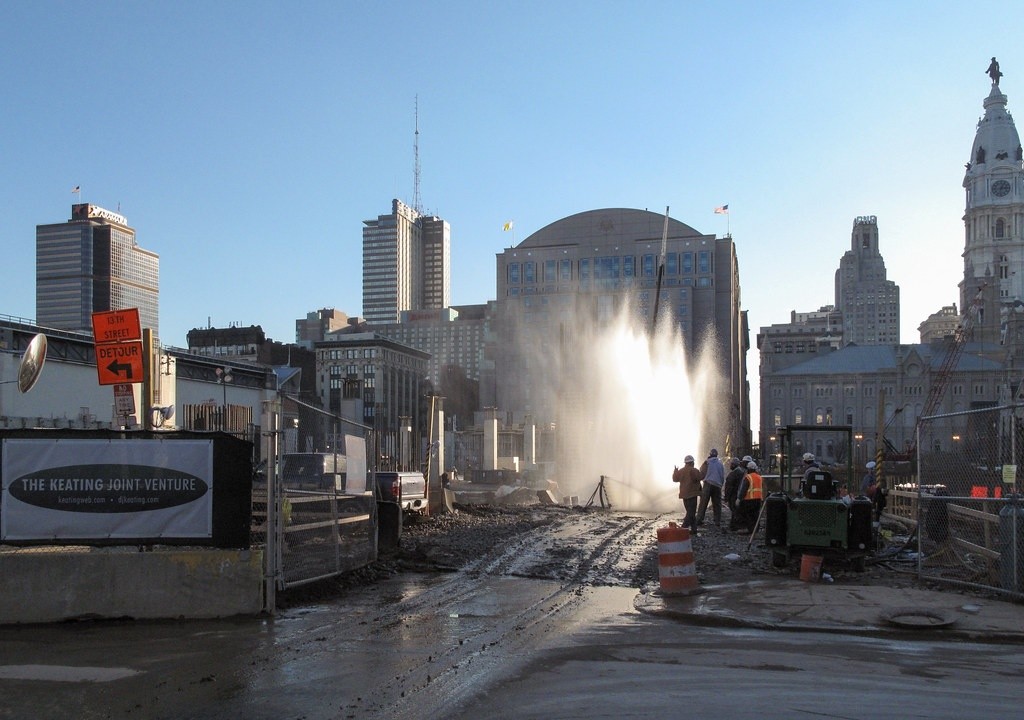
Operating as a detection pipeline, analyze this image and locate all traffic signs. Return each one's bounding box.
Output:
[95,339,145,387]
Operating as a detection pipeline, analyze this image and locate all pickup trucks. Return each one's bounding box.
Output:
[252,452,428,526]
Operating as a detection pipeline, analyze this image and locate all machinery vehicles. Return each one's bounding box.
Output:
[766,422,874,572]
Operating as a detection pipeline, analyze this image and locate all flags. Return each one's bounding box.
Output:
[72,186,80,193]
[504,222,511,231]
[714,205,728,214]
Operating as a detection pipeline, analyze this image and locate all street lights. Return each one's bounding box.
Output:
[214,366,232,405]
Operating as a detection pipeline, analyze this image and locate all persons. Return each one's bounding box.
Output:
[724,456,762,534]
[861,468,875,493]
[802,454,821,484]
[697,449,724,526]
[673,456,709,536]
[985,57,1000,84]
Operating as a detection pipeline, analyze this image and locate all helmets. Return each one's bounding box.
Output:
[684,455,694,462]
[741,456,752,462]
[803,453,815,461]
[747,461,758,470]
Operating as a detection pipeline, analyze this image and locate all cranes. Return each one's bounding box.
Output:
[879,280,988,476]
